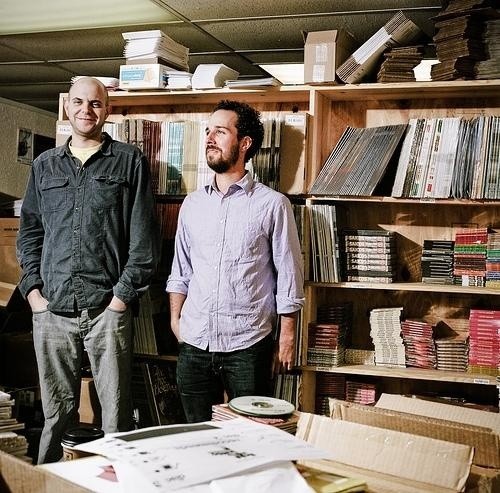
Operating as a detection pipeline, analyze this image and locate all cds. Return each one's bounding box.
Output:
[229,396,295,417]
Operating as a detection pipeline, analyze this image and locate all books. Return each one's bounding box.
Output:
[0,391,33,467]
[266,306,302,411]
[308,204,500,289]
[306,299,500,419]
[308,115,500,200]
[100,118,281,194]
[68,28,282,90]
[129,288,186,426]
[157,201,181,243]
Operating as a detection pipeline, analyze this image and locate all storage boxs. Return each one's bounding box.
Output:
[302,27,362,85]
[119,64,179,92]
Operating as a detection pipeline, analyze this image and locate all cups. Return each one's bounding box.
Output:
[59,426,104,460]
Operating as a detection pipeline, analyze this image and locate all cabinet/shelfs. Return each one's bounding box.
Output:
[56,78,498,434]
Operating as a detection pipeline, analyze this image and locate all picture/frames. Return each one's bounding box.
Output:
[16,125,34,166]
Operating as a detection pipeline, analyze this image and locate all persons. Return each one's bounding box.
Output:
[16,75,160,467]
[165,99,304,421]
